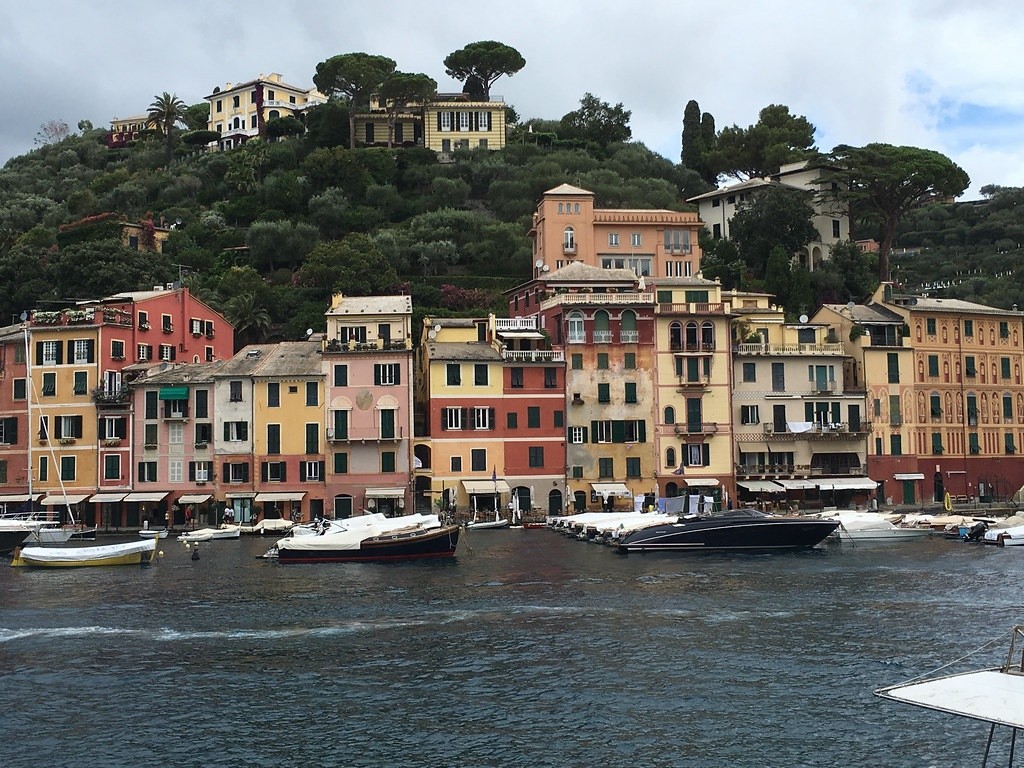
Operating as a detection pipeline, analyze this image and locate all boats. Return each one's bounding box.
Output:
[462,480,1024,552]
[139,528,170,539]
[256,507,460,565]
[176,521,242,542]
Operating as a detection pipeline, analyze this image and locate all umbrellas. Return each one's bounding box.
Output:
[565,484,570,515]
[654,482,660,511]
[449,485,458,513]
[529,485,535,506]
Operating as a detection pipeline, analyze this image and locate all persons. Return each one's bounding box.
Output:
[223,506,235,524]
[269,505,282,519]
[183,506,192,528]
[368,499,375,515]
[164,511,170,533]
[886,494,894,511]
[507,500,513,514]
[728,497,732,510]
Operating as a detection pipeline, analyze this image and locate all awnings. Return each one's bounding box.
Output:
[89,493,130,503]
[178,495,212,504]
[123,492,170,502]
[461,480,511,493]
[365,487,405,499]
[0,494,46,502]
[225,492,257,498]
[683,478,720,486]
[591,484,631,497]
[736,477,879,492]
[414,456,423,469]
[254,492,307,502]
[40,493,92,506]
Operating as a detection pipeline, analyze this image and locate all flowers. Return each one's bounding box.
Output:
[58,439,76,443]
[103,439,122,446]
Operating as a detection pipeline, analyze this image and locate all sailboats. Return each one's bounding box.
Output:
[0,372,161,569]
